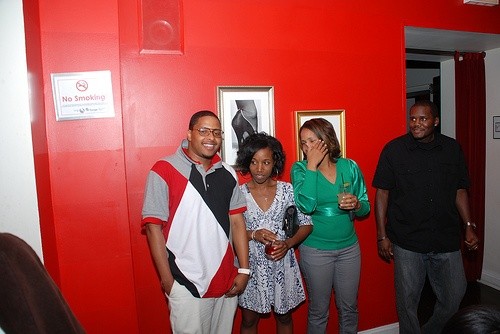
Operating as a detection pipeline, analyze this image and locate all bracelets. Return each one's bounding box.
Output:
[377,235,388,242]
[464,222,476,231]
[252,230,256,241]
[238,269,252,277]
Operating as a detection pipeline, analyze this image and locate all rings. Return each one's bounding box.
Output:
[263,233,266,238]
[351,203,354,206]
[475,246,478,249]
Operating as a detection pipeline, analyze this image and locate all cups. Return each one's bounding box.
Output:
[338,193,352,209]
[265,233,281,260]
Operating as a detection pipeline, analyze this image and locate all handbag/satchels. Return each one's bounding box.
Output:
[282,205,299,238]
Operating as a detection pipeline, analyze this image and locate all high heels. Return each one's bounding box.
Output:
[232,109,257,147]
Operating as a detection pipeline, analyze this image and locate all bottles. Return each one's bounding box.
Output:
[284,206,297,238]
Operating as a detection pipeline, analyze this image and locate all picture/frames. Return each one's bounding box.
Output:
[493,116,500,139]
[217,86,275,170]
[294,109,347,162]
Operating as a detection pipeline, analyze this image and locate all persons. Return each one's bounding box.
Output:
[238,131,313,334]
[372,100,479,334]
[141,110,249,333]
[290,118,370,334]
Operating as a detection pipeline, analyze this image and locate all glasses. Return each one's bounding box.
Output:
[192,128,224,138]
